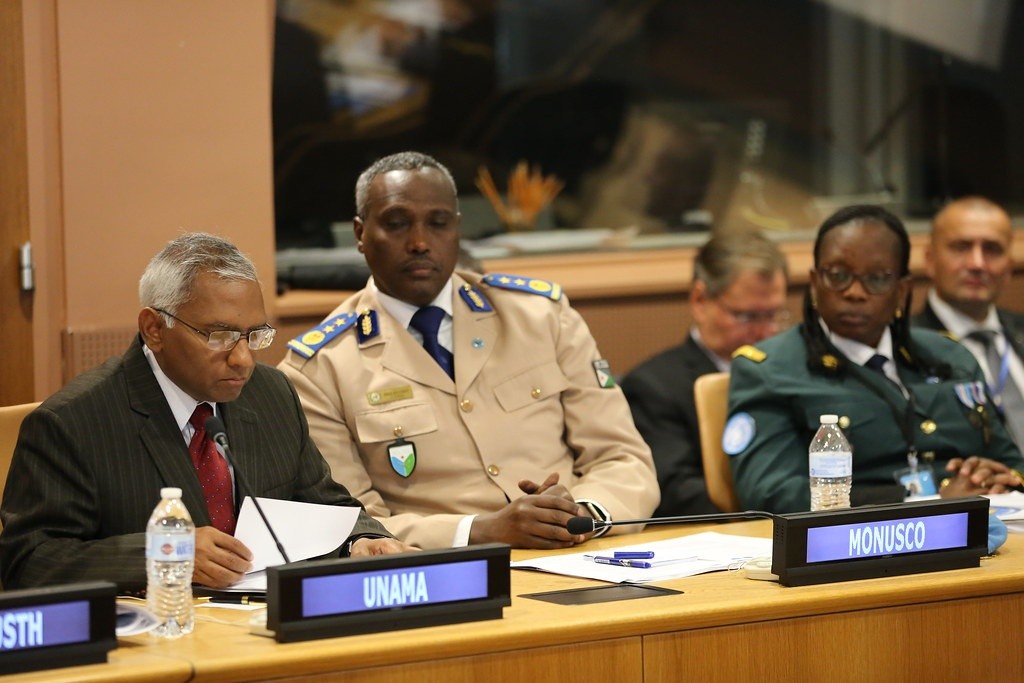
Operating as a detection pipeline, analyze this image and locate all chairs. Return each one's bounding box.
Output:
[694,371,745,522]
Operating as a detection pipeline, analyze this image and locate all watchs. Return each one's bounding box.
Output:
[581,502,605,533]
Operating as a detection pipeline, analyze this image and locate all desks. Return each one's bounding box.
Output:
[0,489,1024,683]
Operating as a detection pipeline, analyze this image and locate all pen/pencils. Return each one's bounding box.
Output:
[584,552,654,559]
[209,594,268,605]
[594,556,651,568]
[474,160,562,230]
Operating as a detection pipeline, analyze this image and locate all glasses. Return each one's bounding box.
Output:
[707,288,790,326]
[151,304,276,351]
[817,265,901,294]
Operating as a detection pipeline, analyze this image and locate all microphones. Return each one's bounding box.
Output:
[203,416,289,563]
[566,510,773,534]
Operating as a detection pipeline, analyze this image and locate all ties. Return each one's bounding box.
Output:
[185,404,235,540]
[866,354,902,396]
[967,329,1024,456]
[411,306,454,381]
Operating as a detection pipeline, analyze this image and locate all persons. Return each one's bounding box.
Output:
[616,226,790,522]
[904,194,1024,459]
[909,484,917,495]
[727,203,1024,514]
[0,232,424,595]
[277,152,660,551]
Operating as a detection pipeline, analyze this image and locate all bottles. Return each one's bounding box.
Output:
[145,487,197,641]
[808,415,853,513]
